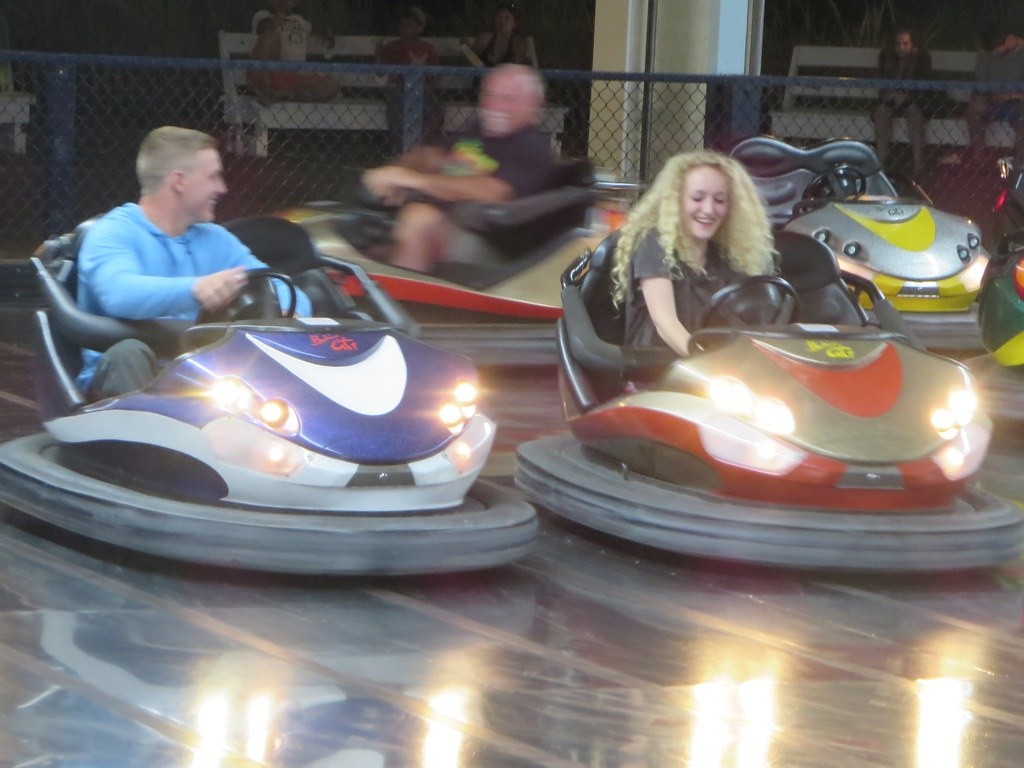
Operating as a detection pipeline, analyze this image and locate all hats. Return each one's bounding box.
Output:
[406,7,426,26]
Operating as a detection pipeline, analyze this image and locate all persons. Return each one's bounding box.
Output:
[610,150,784,393]
[72,125,314,404]
[963,22,1024,174]
[247,0,344,104]
[376,6,445,150]
[471,2,524,71]
[868,26,938,187]
[357,62,589,289]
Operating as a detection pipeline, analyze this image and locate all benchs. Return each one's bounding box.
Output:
[721,133,893,218]
[581,220,869,346]
[28,215,374,385]
[0,43,38,156]
[766,48,1024,147]
[217,31,567,160]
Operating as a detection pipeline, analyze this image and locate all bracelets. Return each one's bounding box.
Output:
[1008,91,1012,100]
